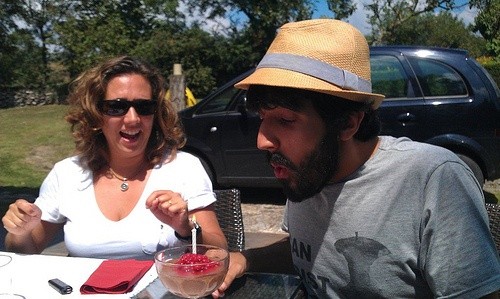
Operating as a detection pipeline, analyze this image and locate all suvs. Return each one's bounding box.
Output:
[176,45,500,193]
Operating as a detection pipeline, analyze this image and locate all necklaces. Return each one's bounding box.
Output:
[107,163,146,191]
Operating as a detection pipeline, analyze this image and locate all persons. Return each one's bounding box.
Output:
[2,54,227,259]
[205,19,500,299]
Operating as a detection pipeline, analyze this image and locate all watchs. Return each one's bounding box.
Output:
[174,220,201,242]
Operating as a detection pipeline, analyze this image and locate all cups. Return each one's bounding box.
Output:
[0,254,13,295]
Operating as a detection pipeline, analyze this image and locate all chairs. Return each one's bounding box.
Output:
[211,188,245,256]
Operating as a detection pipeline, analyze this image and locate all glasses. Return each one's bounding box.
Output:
[99,98,159,117]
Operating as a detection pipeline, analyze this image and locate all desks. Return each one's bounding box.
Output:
[0,249,302,299]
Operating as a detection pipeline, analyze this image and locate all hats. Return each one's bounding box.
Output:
[233,18,385,111]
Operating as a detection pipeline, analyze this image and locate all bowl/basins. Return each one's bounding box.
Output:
[154,244,230,299]
